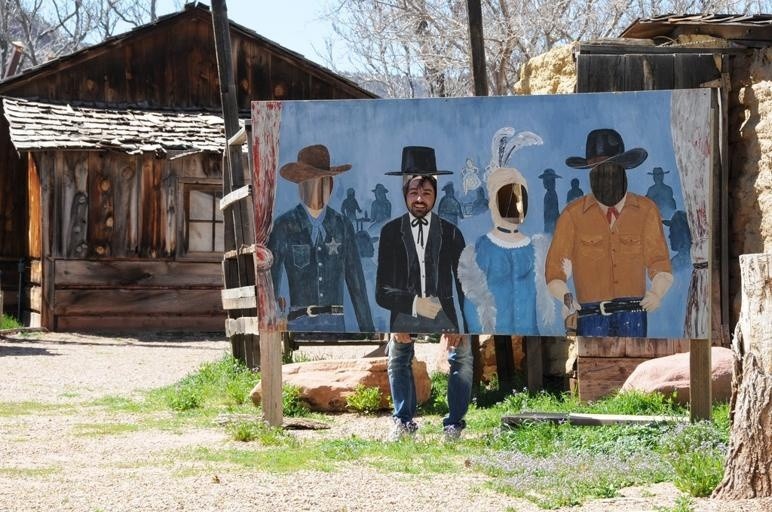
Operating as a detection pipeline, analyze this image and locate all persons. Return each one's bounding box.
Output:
[388,176,474,445]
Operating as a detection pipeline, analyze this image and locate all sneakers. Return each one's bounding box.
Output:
[442,424,462,443]
[396,419,419,436]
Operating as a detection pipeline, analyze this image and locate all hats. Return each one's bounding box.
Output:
[280,146,351,185]
[538,169,562,178]
[663,211,687,226]
[565,129,648,170]
[442,183,453,192]
[486,127,543,231]
[372,184,389,193]
[384,146,454,176]
[648,168,670,175]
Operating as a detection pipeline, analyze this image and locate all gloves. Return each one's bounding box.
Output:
[548,280,582,318]
[639,271,674,314]
[561,257,572,279]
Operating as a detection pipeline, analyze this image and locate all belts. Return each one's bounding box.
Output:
[578,299,644,318]
[287,304,345,321]
